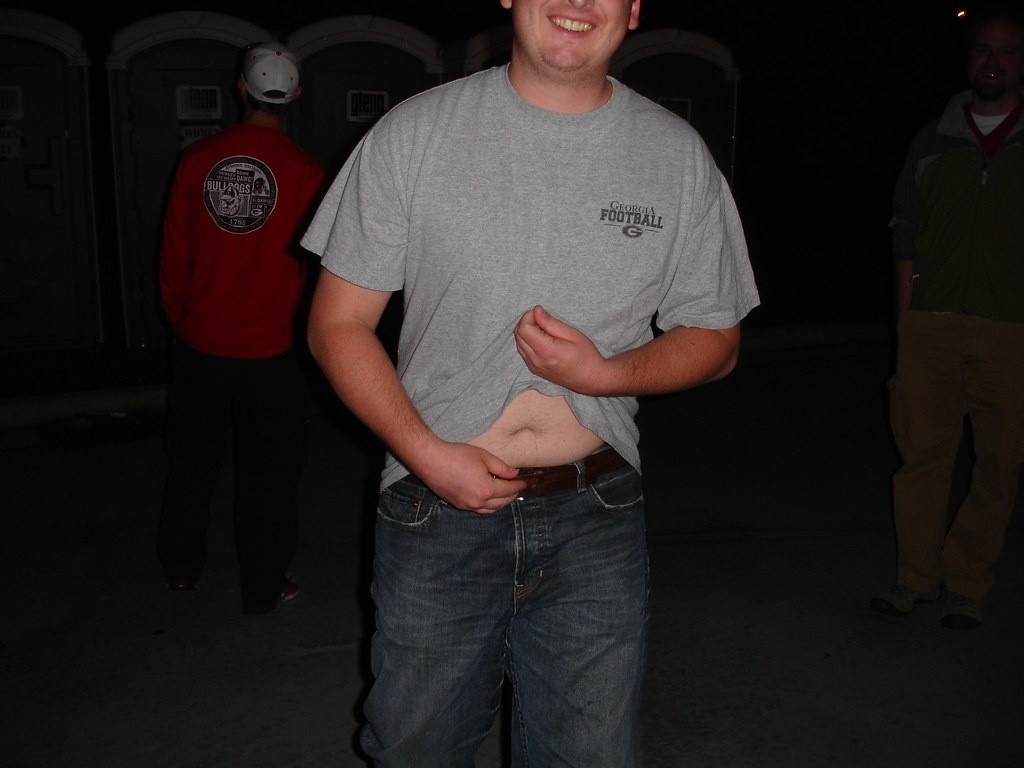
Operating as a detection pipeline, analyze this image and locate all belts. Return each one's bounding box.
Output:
[401,449,628,501]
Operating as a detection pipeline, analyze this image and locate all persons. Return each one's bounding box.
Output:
[154,43,325,614]
[301,0,764,768]
[869,16,1024,627]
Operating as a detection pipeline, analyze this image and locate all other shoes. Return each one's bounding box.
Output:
[870,581,946,617]
[280,573,300,602]
[156,560,198,592]
[940,580,984,630]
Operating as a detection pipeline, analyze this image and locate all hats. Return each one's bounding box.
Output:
[241,40,299,104]
